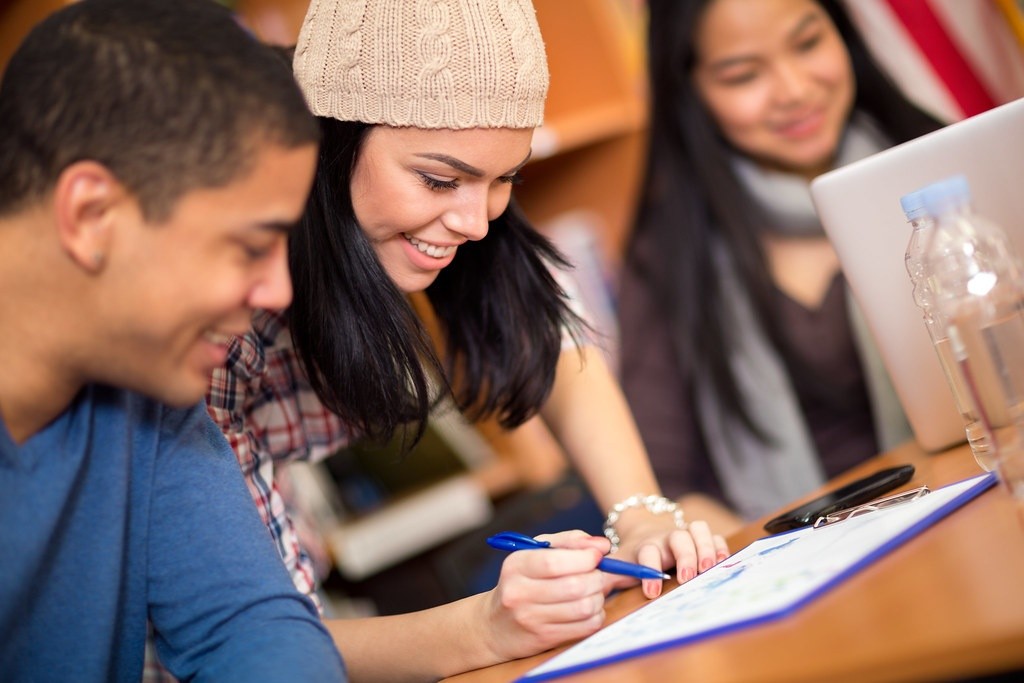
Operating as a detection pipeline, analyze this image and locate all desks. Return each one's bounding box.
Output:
[437,423,1023,682]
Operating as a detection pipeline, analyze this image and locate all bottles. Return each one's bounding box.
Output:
[898,175,1024,518]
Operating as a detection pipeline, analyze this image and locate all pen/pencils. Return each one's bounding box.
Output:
[486,529,673,583]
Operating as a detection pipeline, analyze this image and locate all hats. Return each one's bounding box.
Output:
[293,0,550,131]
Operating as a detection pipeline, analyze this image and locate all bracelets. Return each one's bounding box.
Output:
[603,495,685,553]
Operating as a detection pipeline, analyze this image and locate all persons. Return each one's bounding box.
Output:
[142,0,730,683]
[0,0,350,683]
[619,0,950,539]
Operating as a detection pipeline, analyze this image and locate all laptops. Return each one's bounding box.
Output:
[809,96,1023,455]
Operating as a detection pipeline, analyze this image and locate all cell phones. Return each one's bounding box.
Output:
[764,465,914,533]
